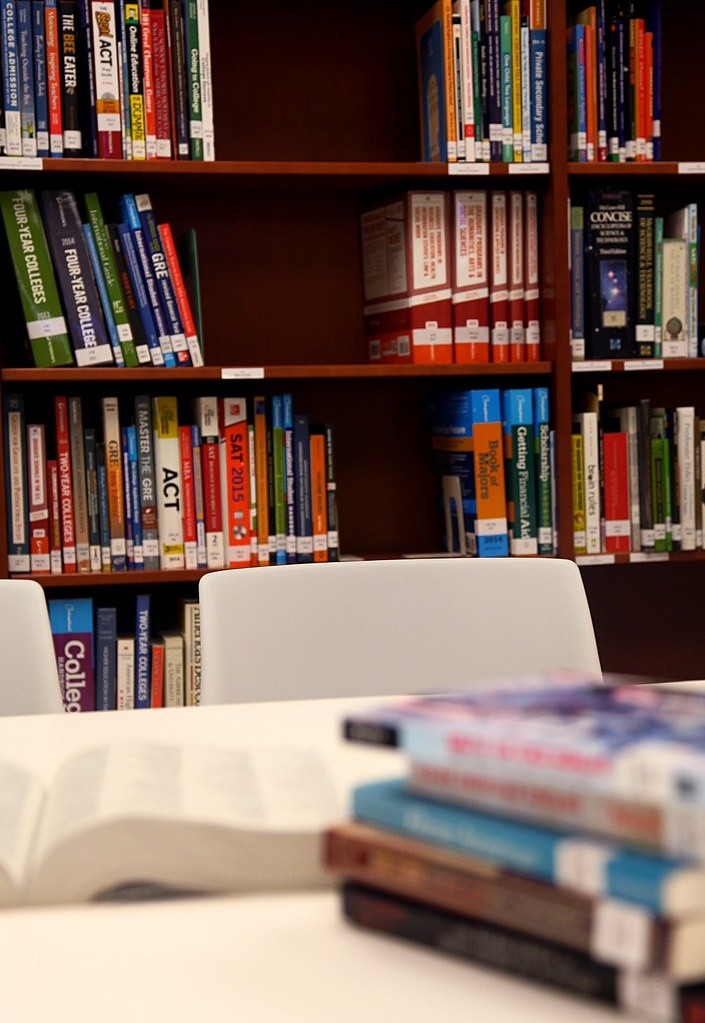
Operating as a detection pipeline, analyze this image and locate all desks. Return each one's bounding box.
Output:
[0,680,705,1023]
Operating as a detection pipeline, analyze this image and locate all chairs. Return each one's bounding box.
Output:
[0,579,66,717]
[198,558,604,708]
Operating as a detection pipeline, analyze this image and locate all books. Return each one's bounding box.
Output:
[321,676,705,1023]
[2,707,355,908]
[0,0,705,576]
[49,593,202,713]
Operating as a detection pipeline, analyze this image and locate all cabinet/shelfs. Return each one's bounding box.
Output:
[0,0,705,588]
